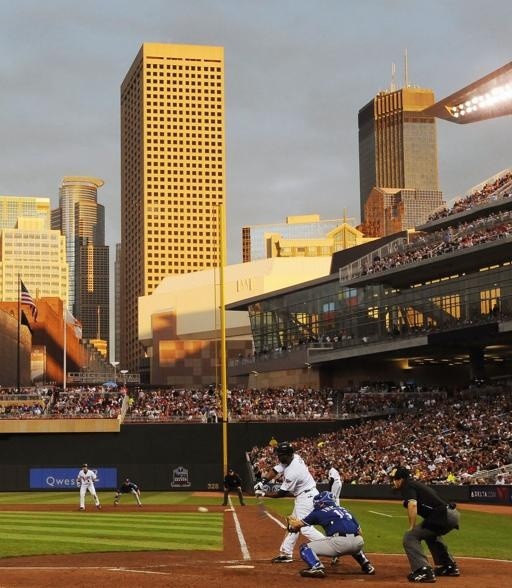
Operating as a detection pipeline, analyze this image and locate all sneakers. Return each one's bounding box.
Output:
[300,568,326,577]
[434,563,459,576]
[363,563,375,575]
[407,566,436,582]
[272,555,293,563]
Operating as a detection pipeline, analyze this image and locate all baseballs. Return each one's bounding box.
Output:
[199,507,207,512]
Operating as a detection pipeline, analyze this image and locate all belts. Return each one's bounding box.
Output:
[339,532,358,537]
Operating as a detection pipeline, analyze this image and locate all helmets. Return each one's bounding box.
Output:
[388,466,409,479]
[276,443,294,458]
[313,491,336,509]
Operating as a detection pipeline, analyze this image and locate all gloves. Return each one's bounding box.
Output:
[253,480,265,490]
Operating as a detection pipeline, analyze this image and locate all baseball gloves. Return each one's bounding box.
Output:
[286,514,299,533]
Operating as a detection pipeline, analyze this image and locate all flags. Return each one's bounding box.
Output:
[63,306,81,335]
[20,280,37,323]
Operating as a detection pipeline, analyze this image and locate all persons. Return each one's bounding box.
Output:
[221,468,246,506]
[326,461,343,500]
[390,467,463,582]
[237,308,512,364]
[249,390,512,485]
[287,490,375,576]
[113,477,143,507]
[354,173,512,277]
[1,380,448,421]
[77,461,101,509]
[254,442,340,565]
